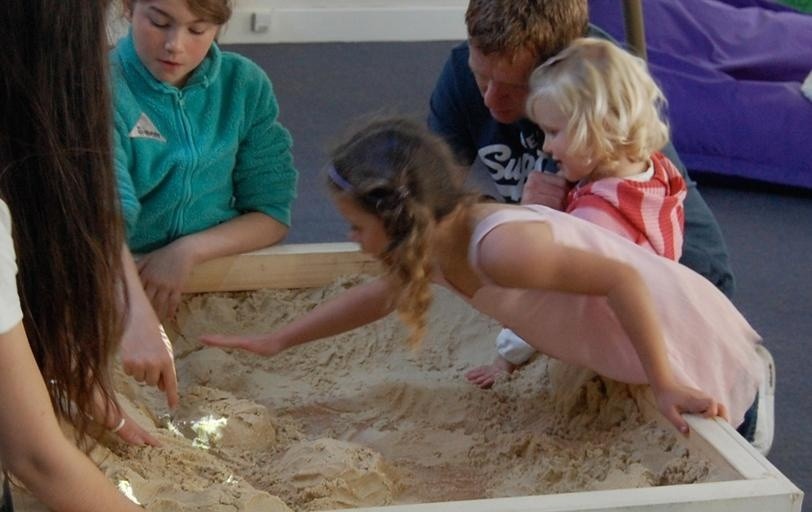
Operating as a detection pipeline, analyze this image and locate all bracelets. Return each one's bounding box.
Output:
[111,418,125,435]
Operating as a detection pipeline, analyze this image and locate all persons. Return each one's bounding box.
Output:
[198,120,777,457]
[0,2,159,511]
[466,39,687,389]
[75,0,300,401]
[429,2,735,295]
